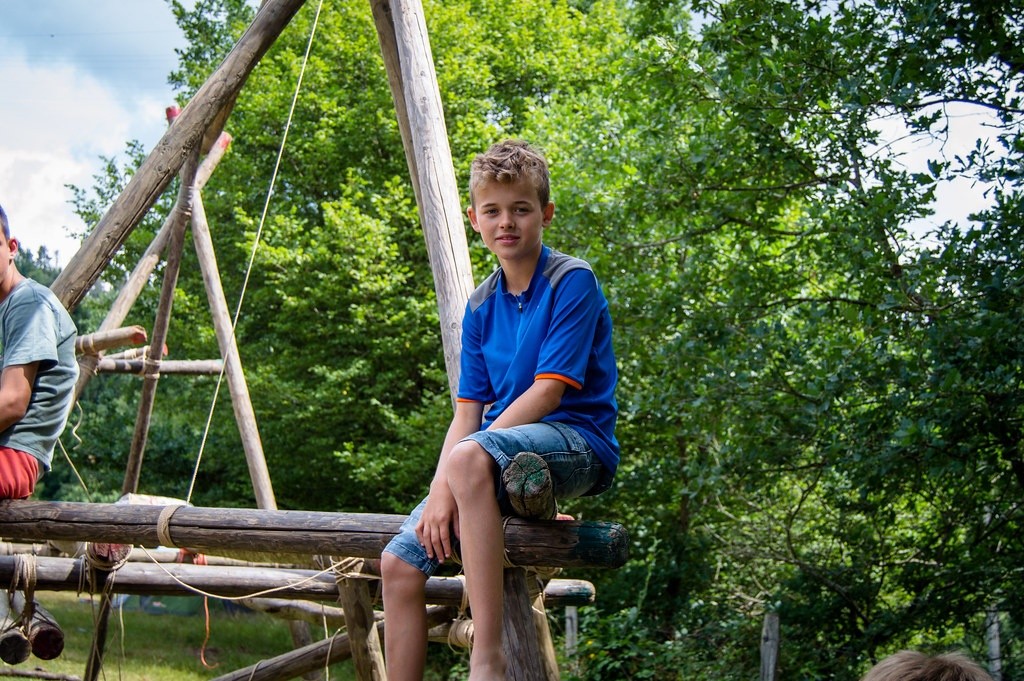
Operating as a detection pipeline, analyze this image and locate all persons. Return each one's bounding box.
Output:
[381,138,620,681]
[861,651,993,681]
[0,205,78,500]
